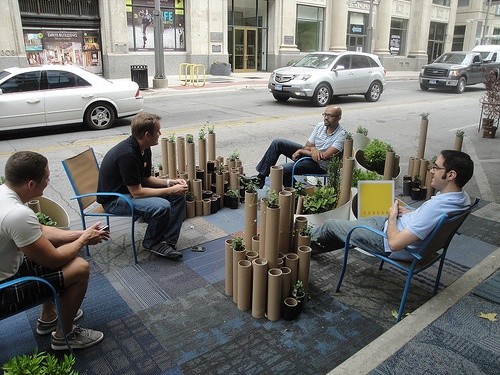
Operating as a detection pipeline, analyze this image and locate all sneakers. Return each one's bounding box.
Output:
[51,324,104,350]
[149,241,183,261]
[240,175,261,189]
[37,309,83,335]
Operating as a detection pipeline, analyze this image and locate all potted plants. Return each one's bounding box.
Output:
[24,111,466,321]
[479,68,500,139]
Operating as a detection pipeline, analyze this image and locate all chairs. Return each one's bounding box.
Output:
[285,149,344,189]
[337,197,480,321]
[0,275,72,354]
[60,147,140,267]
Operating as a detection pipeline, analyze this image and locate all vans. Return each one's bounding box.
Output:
[472,45,500,64]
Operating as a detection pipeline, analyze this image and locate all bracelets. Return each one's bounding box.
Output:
[166,179,170,187]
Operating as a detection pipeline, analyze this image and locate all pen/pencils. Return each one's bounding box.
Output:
[99,225,109,231]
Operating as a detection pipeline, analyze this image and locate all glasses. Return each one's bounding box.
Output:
[322,113,338,117]
[431,165,445,171]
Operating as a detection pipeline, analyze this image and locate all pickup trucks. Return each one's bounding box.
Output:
[419,50,500,95]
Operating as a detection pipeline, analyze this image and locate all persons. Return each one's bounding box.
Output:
[95,112,189,259]
[240,106,348,189]
[0,151,110,350]
[311,150,474,262]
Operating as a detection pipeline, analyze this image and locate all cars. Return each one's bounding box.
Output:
[0,64,145,131]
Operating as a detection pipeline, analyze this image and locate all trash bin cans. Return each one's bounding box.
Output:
[130,64,148,90]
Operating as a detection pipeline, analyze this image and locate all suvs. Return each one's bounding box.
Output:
[268,50,388,108]
